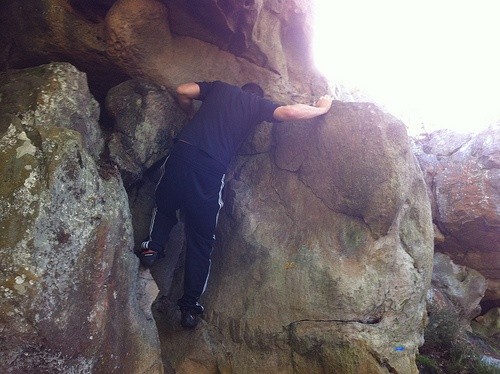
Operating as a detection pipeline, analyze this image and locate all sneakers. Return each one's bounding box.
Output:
[140,252,162,267]
[181,310,198,328]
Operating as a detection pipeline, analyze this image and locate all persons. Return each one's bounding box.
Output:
[139,81,333,327]
